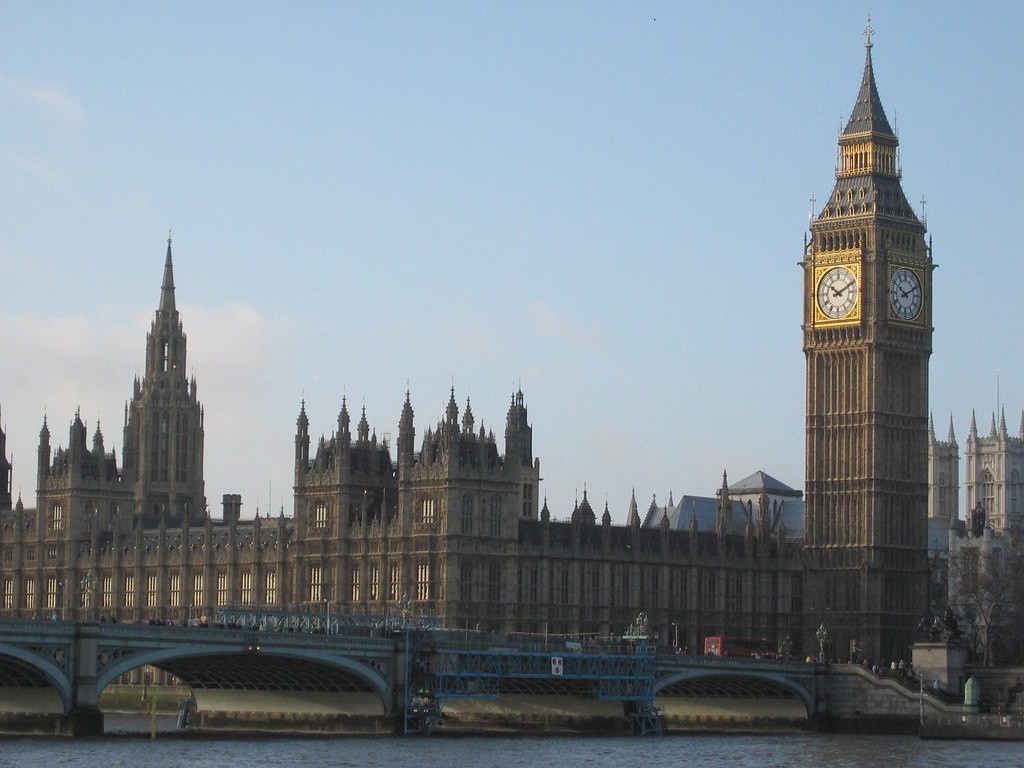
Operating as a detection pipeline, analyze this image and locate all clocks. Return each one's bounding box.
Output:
[888,264,925,324]
[814,264,859,322]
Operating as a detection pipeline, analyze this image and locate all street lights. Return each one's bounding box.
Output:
[672,622,678,653]
[324,598,329,635]
[188,604,193,627]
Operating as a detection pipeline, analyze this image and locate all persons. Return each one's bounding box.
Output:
[864,659,906,674]
[148,614,165,625]
[677,646,689,654]
[942,605,953,629]
[703,650,785,658]
[100,612,115,623]
[52,608,61,621]
